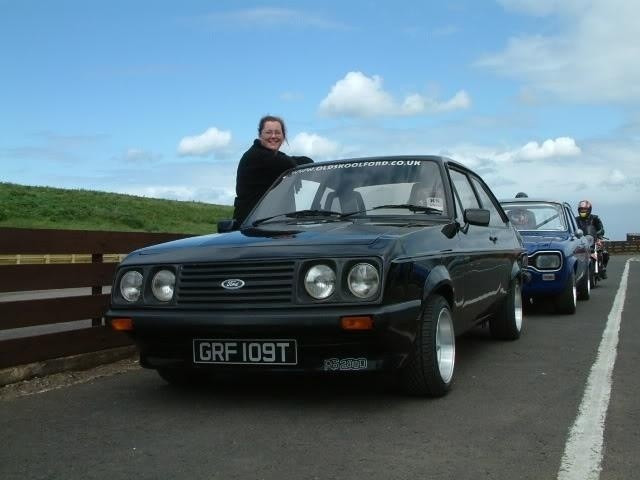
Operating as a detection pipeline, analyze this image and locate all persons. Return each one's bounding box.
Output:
[575,200,609,279]
[507,193,536,231]
[233,116,313,230]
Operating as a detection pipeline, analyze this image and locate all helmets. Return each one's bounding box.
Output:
[578,200,592,218]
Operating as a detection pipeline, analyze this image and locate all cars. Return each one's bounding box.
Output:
[495,198,595,313]
[107,153,535,397]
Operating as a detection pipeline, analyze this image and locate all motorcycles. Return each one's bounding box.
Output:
[580,227,608,288]
